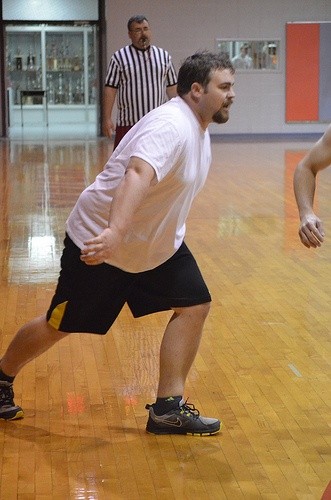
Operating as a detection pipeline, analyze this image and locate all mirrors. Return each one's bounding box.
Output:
[215,37,282,72]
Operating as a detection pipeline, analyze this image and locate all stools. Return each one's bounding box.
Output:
[19,89,48,127]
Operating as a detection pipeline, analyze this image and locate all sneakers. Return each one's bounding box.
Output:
[0,381,24,420]
[145,396,222,436]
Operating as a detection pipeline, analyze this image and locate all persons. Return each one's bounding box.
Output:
[0,53,236,436]
[102,14,179,152]
[293,123,331,249]
[230,43,254,70]
